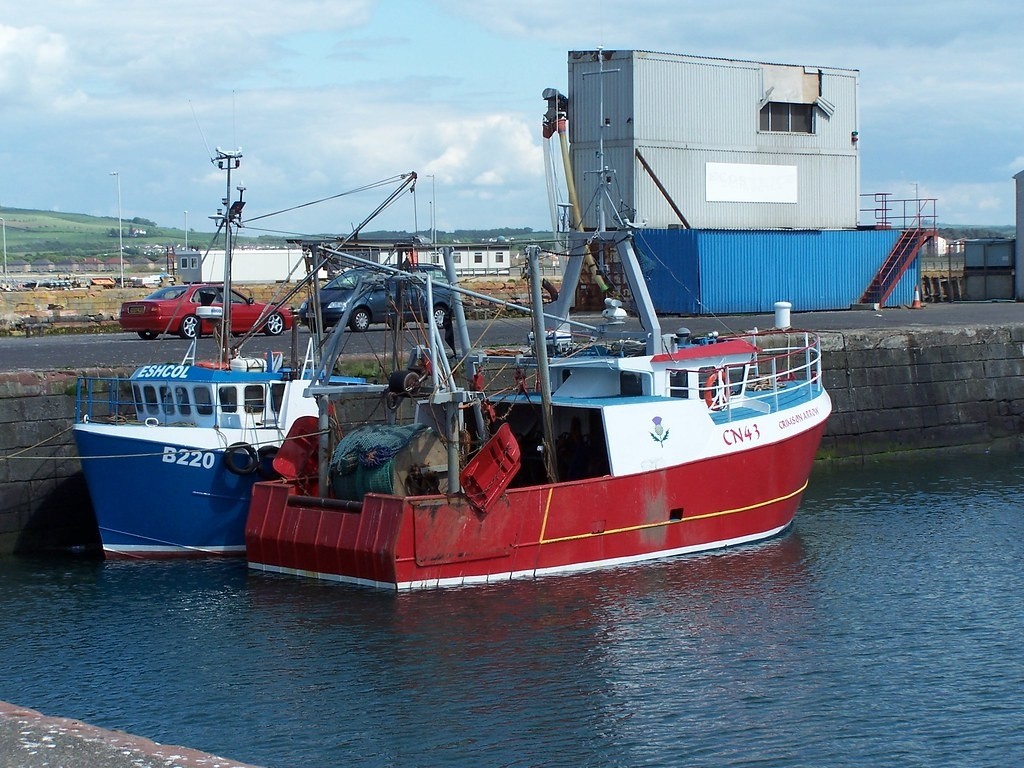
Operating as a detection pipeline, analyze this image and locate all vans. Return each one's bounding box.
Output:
[300,263,457,330]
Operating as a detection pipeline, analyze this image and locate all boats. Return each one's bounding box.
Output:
[241,220,838,596]
[71,45,735,562]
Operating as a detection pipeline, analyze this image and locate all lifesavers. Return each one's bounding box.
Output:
[703,371,732,412]
[194,361,228,370]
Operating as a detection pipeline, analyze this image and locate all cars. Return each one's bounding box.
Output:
[121,284,294,338]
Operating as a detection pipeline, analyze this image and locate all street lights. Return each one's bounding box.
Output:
[181,210,190,251]
[424,173,438,268]
[109,170,125,290]
[0,216,10,286]
